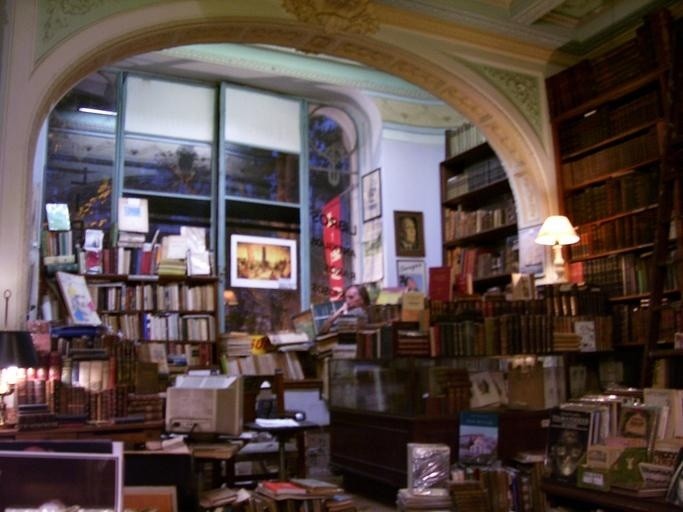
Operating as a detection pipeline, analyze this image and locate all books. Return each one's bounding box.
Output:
[355,275,682,510]
[442,123,519,303]
[544,35,683,286]
[0,227,354,512]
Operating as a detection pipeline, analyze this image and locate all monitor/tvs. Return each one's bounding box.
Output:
[167,374,243,439]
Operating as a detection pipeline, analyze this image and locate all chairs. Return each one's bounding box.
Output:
[220,368,305,481]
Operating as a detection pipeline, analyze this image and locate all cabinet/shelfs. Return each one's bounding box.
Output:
[36,270,222,396]
[427,0,682,417]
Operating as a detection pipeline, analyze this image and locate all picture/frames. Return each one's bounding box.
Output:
[230,234,300,294]
[393,209,426,298]
[361,167,382,223]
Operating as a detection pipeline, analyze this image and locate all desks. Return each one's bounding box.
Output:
[125,434,249,502]
